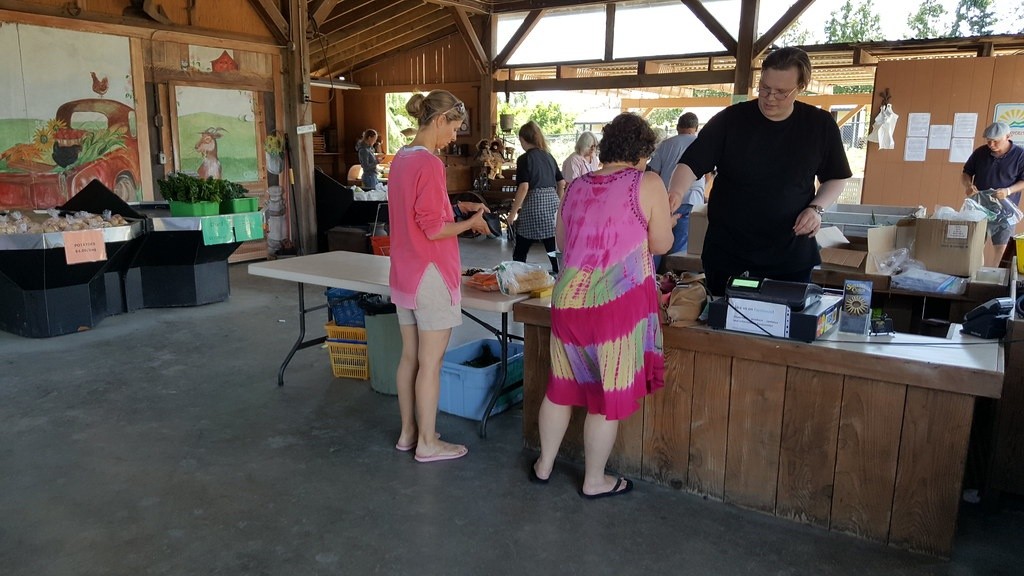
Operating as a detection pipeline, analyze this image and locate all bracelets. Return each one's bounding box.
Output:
[1007,188,1010,195]
[453,204,462,216]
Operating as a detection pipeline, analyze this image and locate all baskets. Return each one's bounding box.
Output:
[325,288,369,380]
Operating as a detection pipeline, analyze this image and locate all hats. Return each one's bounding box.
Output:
[983,122,1010,139]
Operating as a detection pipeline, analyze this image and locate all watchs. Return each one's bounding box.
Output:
[808,205,824,217]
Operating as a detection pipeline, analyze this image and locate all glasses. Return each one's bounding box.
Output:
[443,102,465,115]
[588,144,596,148]
[756,79,802,99]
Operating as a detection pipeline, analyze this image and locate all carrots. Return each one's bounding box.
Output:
[471,272,499,287]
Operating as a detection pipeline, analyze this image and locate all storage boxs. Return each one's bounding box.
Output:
[814,216,989,274]
[221,197,260,214]
[438,338,526,421]
[169,200,220,217]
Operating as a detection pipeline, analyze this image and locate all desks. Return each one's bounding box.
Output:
[248,250,532,439]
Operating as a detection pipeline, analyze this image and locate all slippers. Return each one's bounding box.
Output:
[415,444,468,462]
[529,456,549,484]
[578,477,633,498]
[396,433,441,450]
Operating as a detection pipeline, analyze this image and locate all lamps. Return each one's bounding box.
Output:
[308,78,362,92]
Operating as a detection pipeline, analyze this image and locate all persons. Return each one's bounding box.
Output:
[562,132,599,191]
[648,112,705,273]
[651,127,667,157]
[961,123,1024,267]
[388,89,491,462]
[528,114,682,499]
[668,47,854,283]
[507,121,565,273]
[704,171,716,204]
[356,129,386,189]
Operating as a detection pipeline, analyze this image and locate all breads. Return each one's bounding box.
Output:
[508,270,554,295]
[0,213,128,235]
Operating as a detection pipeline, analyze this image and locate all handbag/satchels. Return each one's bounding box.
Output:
[666,272,707,327]
[461,211,502,239]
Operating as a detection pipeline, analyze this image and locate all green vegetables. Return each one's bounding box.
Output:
[461,268,485,276]
[157,173,249,204]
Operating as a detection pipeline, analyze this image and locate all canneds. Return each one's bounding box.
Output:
[511,175,516,180]
[502,186,517,192]
